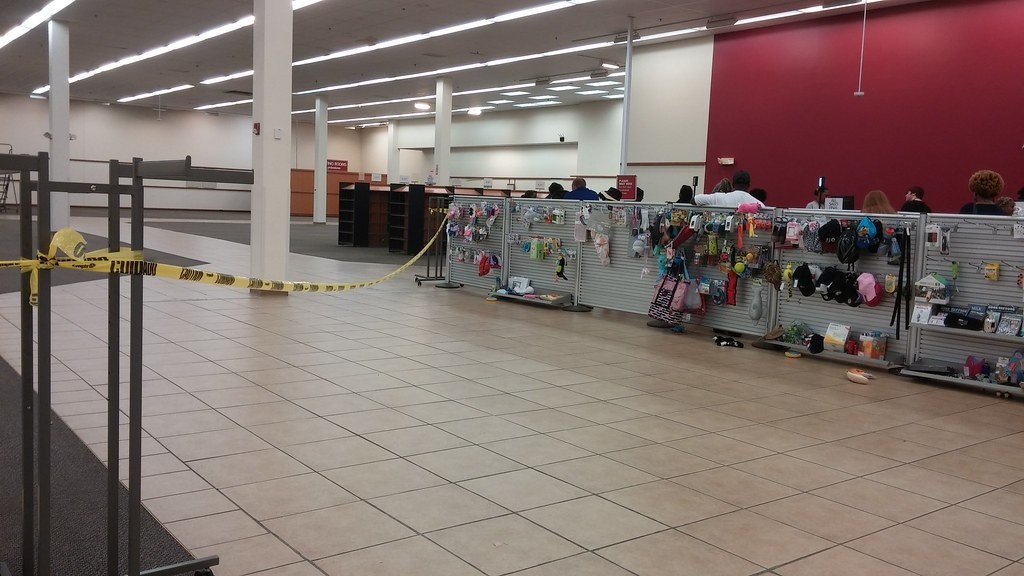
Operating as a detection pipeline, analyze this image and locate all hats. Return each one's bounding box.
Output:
[598,187,622,201]
[793,262,882,307]
[819,216,883,271]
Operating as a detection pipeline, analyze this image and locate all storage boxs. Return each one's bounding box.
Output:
[823,323,851,352]
[860,336,887,359]
[914,273,950,305]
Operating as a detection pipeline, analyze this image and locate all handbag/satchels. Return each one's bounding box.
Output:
[648,257,705,326]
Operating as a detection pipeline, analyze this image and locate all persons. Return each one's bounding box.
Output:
[676,185,693,203]
[521,190,537,198]
[690,171,765,207]
[898,187,932,213]
[806,187,829,209]
[996,196,1015,216]
[565,178,599,200]
[861,190,896,214]
[749,188,767,202]
[959,170,1007,216]
[545,182,568,199]
[712,178,733,195]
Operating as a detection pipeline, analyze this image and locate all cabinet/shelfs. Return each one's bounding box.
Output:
[435,194,1024,400]
[290,167,551,257]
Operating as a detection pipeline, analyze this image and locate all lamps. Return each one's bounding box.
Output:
[822,0,861,8]
[706,17,739,29]
[205,111,218,116]
[535,79,552,86]
[614,32,640,43]
[589,70,611,78]
